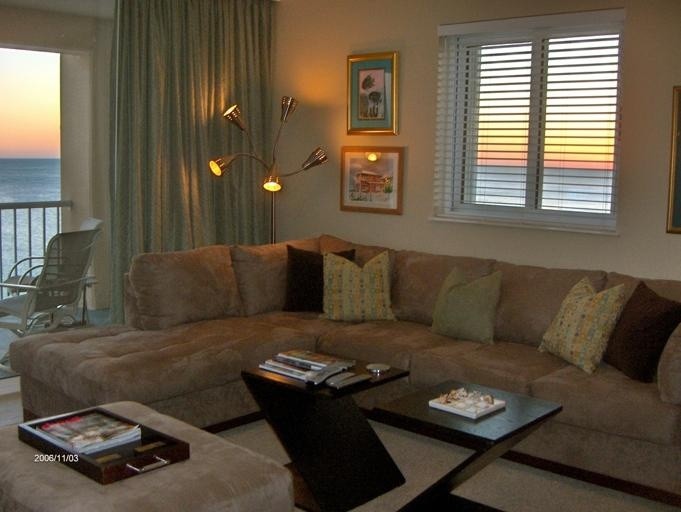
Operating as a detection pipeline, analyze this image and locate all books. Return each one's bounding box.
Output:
[36,411,142,455]
[258,349,355,385]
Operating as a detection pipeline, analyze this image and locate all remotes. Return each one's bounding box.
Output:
[333,374,372,389]
[304,366,342,386]
[326,372,355,387]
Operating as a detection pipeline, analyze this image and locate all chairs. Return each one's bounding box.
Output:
[3,215,103,327]
[1,229,99,336]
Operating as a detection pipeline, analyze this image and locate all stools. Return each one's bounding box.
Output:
[3,400,296,508]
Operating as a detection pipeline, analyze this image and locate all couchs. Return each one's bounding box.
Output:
[9,233,681,496]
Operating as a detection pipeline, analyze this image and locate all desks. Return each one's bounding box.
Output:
[360,378,564,510]
[240,352,413,511]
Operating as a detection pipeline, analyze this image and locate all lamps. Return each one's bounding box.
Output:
[207,95,329,244]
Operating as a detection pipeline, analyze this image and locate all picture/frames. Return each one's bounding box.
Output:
[340,144,408,217]
[669,85,681,233]
[346,52,402,136]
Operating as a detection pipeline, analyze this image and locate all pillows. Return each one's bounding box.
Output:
[430,266,502,347]
[284,241,356,314]
[605,281,681,383]
[319,248,399,321]
[541,278,626,374]
